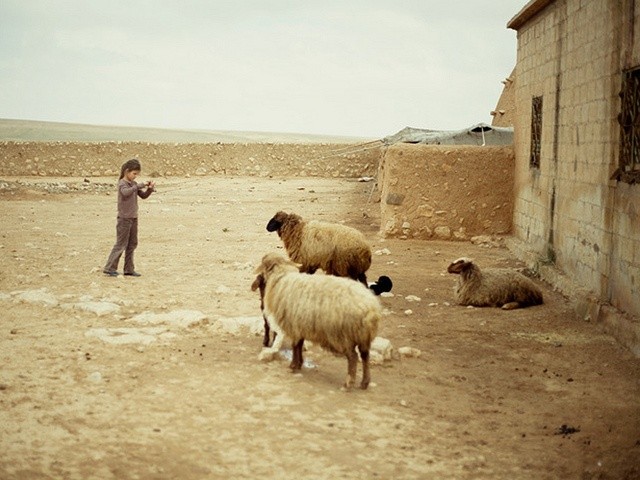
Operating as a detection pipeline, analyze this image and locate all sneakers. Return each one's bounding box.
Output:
[124,272,141,276]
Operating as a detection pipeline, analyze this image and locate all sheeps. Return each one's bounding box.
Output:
[252,250,381,392]
[266,211,372,288]
[447,256,543,309]
[251,272,281,348]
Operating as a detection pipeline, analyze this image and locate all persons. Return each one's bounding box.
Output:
[101,159,156,278]
